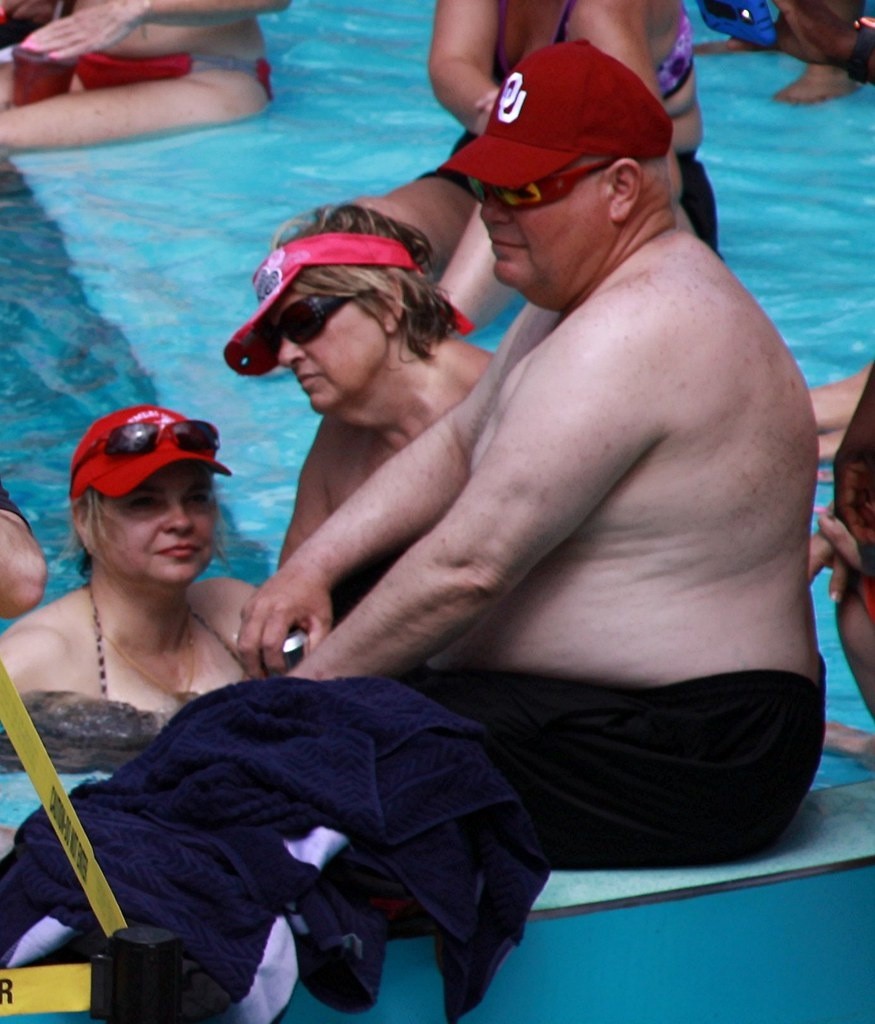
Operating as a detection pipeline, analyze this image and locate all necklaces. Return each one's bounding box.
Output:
[83,582,201,703]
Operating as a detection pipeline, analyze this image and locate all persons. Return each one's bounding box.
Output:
[688,0,866,104]
[348,0,720,338]
[223,205,498,577]
[834,364,875,545]
[0,0,292,154]
[806,364,872,465]
[1,483,48,620]
[238,32,826,877]
[0,405,262,715]
[808,501,875,770]
[725,0,875,86]
[0,0,55,49]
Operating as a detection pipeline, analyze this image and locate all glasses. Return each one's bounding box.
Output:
[464,157,624,209]
[69,420,221,495]
[264,293,358,352]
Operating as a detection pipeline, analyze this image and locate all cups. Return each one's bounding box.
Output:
[11,50,77,108]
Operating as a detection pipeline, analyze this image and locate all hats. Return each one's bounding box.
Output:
[71,404,232,499]
[440,37,674,190]
[222,233,475,376]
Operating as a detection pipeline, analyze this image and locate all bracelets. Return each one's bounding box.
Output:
[849,26,875,84]
